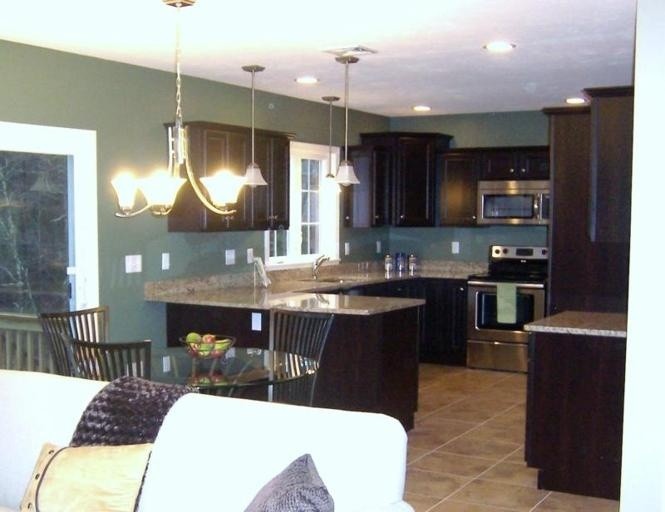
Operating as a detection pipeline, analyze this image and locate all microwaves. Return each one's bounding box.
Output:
[476,180,552,226]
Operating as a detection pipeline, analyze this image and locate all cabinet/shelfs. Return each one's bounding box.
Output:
[163,119,250,234]
[343,145,388,228]
[363,136,449,226]
[247,128,292,231]
[365,280,418,299]
[521,331,621,480]
[483,147,550,180]
[580,85,631,247]
[415,277,468,364]
[436,151,482,227]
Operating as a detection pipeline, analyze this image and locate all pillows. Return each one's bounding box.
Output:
[244,454,336,511]
[17,440,154,512]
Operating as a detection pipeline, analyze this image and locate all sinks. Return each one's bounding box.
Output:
[297,278,367,283]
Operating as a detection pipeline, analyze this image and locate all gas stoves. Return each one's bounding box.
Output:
[468,245,548,285]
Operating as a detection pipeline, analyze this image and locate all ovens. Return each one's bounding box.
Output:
[467,279,547,344]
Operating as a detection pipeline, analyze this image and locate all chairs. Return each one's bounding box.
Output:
[35,306,335,408]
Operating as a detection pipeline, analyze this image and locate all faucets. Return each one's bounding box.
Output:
[313,254,330,279]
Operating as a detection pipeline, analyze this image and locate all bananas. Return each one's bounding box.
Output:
[213,343,231,350]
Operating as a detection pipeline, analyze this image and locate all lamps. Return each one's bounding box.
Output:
[107,0,268,220]
[318,53,363,194]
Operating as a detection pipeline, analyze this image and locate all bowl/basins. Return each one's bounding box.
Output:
[178,334,236,360]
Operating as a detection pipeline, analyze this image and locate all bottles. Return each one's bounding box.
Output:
[384,254,393,279]
[395,252,406,275]
[407,254,416,276]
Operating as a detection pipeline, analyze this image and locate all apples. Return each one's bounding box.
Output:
[210,350,224,358]
[186,333,202,345]
[202,334,215,344]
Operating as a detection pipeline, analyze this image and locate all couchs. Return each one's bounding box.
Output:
[0,366,418,512]
